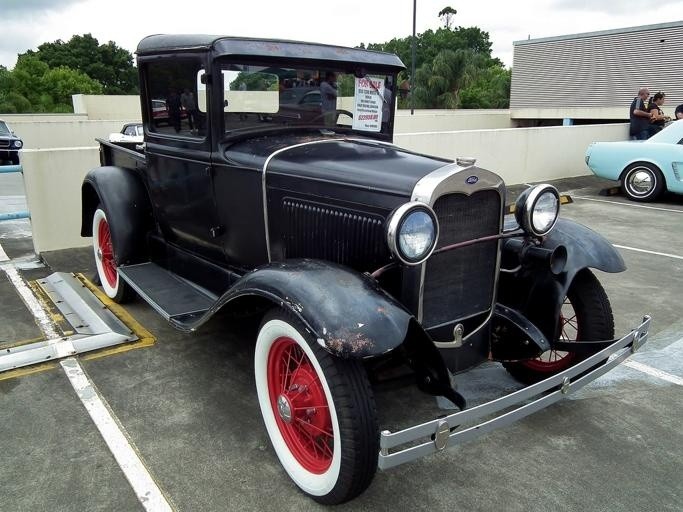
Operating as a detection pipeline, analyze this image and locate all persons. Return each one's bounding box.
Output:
[629,87,656,140]
[646,91,672,137]
[320,71,340,129]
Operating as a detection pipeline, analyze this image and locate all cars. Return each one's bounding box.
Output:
[0,118,23,166]
[584,117,683,204]
[75,29,649,506]
